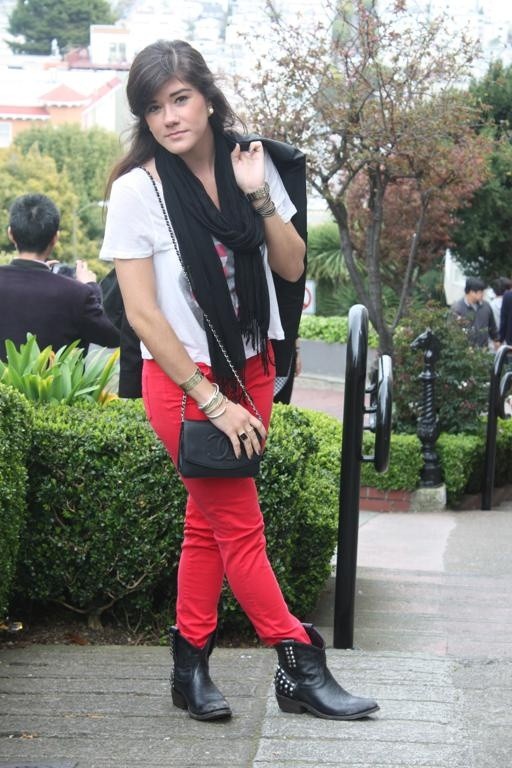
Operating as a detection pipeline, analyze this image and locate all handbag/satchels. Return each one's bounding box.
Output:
[177,420,261,477]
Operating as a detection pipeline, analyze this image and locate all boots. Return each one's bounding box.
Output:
[273,623,380,719]
[170,626,233,720]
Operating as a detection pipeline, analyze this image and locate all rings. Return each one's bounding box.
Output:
[239,434,246,440]
[247,427,253,430]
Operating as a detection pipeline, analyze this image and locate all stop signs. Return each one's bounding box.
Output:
[301,278,317,315]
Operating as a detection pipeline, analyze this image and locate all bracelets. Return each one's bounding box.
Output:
[247,182,276,216]
[180,368,203,391]
[197,382,228,419]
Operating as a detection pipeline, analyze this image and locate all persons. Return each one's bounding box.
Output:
[449,277,512,419]
[85,268,142,398]
[0,193,120,364]
[98,39,381,721]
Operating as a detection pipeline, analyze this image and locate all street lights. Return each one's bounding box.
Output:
[72,199,110,269]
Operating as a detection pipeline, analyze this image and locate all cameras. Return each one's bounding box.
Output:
[50,263,77,281]
